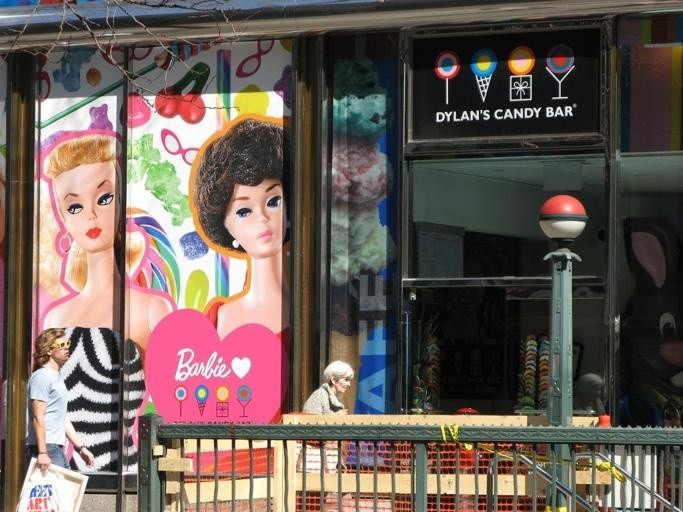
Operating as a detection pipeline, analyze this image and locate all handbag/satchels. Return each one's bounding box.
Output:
[296,446,348,475]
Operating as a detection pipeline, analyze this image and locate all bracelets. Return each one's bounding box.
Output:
[37,452,46,455]
[74,445,85,453]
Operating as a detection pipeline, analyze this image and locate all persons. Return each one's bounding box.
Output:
[37,127,178,475]
[28,328,96,511]
[186,108,296,423]
[574,373,607,417]
[433,284,490,343]
[299,359,356,466]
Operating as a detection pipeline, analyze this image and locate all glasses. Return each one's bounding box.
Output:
[50,340,71,348]
